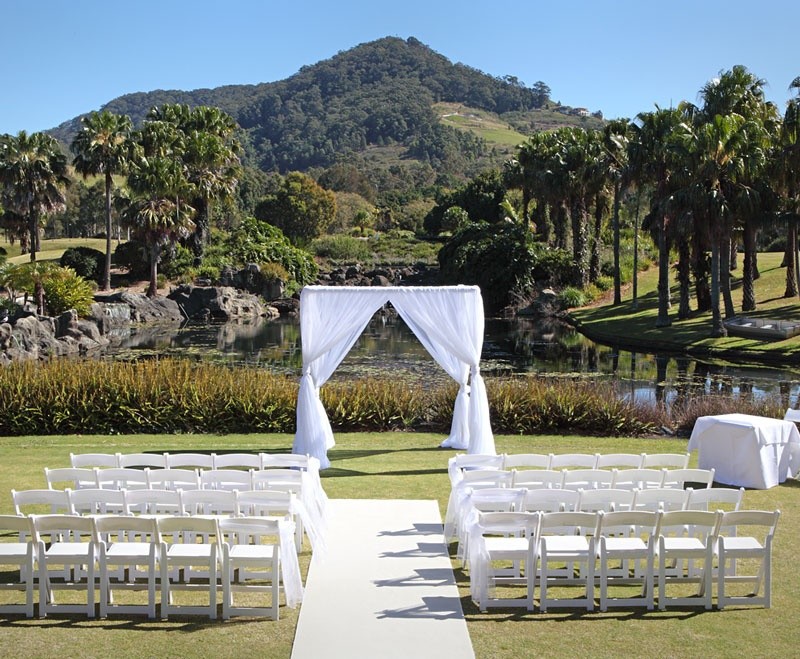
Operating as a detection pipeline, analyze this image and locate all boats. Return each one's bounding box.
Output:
[724,316,800,341]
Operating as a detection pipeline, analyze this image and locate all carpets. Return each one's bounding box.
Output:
[290,499,476,659]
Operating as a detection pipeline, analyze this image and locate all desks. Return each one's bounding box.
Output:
[687,413,800,490]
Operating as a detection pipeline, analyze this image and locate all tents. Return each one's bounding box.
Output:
[291,284,499,471]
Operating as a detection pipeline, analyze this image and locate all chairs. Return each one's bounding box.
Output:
[444,452,780,614]
[0,452,328,621]
